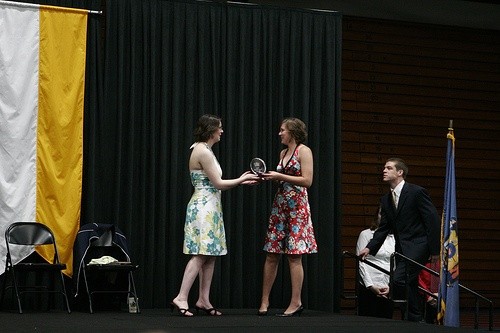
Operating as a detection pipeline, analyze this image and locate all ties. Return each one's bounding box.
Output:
[393,191,398,210]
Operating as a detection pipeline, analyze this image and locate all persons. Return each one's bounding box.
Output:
[355,200,401,319]
[257,118,319,316]
[172,115,260,316]
[417,255,447,322]
[359,157,443,325]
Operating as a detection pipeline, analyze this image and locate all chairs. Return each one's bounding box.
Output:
[76,222,140,313]
[2,222,70,313]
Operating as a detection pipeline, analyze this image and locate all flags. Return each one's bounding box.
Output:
[437,129,461,328]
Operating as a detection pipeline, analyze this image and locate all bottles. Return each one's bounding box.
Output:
[128,289,137,313]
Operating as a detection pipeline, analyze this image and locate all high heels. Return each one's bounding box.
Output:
[170,301,194,317]
[196,304,224,317]
[277,305,304,317]
[258,306,270,316]
